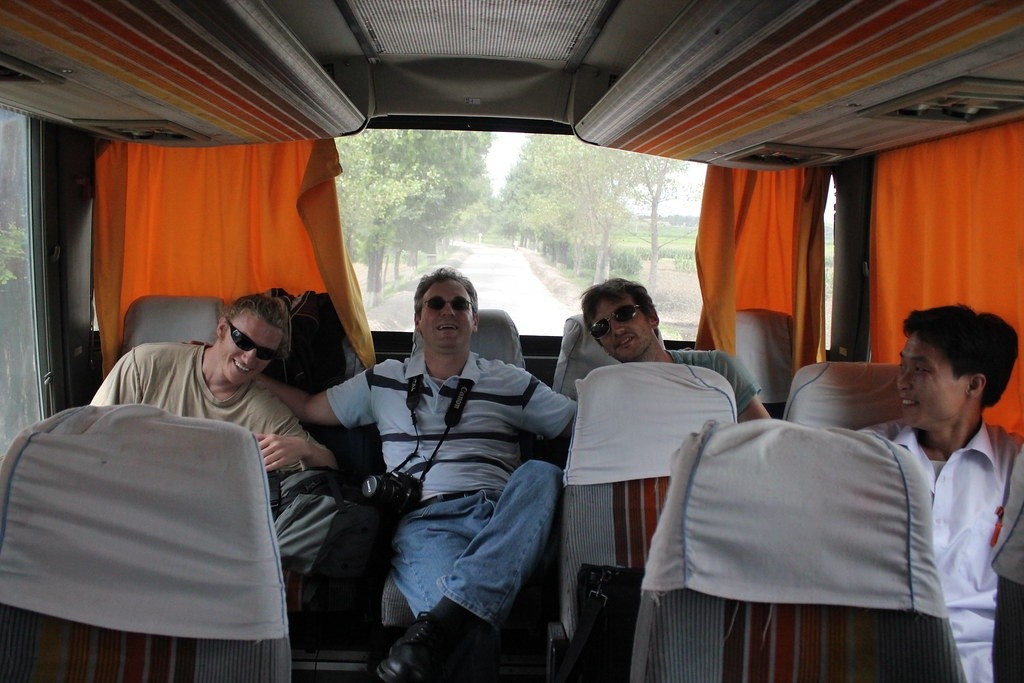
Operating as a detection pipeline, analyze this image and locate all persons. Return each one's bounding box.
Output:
[580,278,772,423]
[183,268,578,683]
[857,303,1024,683]
[90,293,337,473]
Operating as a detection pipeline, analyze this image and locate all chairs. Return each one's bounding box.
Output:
[782,362,904,430]
[411,313,525,375]
[122,295,224,357]
[632,419,967,683]
[547,362,737,683]
[735,309,794,419]
[552,319,667,401]
[0,404,291,683]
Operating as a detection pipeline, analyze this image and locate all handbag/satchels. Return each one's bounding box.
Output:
[274,468,382,582]
[555,564,645,683]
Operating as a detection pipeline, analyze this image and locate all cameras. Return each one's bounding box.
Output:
[361,469,422,515]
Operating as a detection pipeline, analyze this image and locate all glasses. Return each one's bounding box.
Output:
[422,296,473,310]
[228,320,276,360]
[589,304,640,339]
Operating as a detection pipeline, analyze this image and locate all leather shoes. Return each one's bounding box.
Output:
[377,610,454,683]
